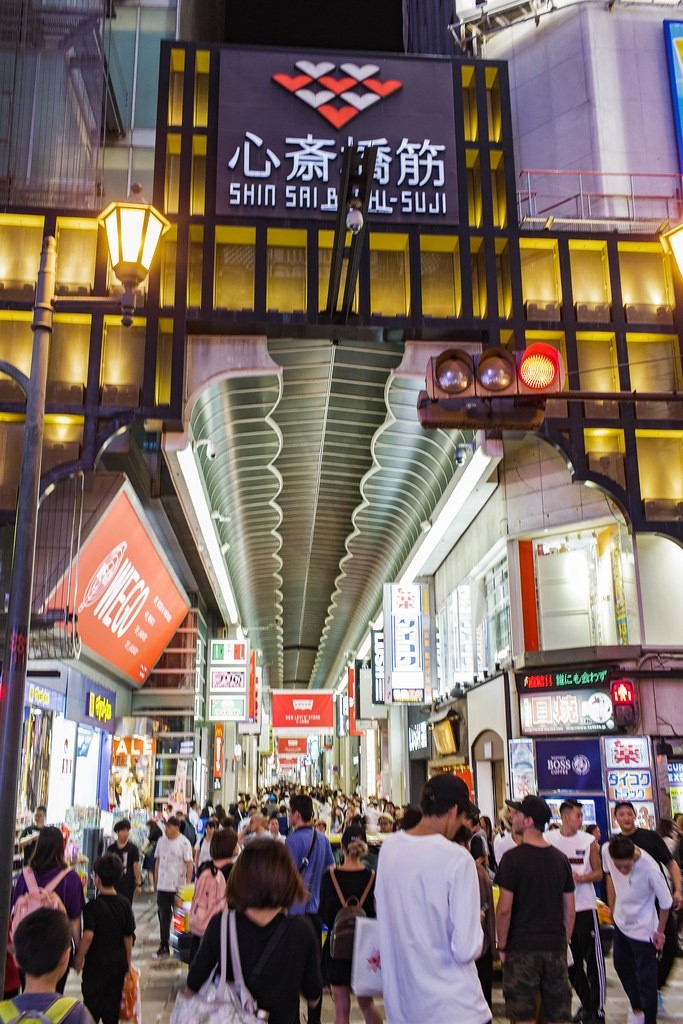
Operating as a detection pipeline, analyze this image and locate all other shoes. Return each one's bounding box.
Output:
[656,990,667,1016]
[626,1011,645,1024]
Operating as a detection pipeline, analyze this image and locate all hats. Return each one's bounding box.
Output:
[505,795,552,824]
[343,826,368,843]
[315,819,327,827]
[378,812,393,824]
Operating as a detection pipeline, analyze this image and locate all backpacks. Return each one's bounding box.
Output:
[188,866,226,937]
[330,868,375,960]
[8,864,73,968]
[0,997,81,1024]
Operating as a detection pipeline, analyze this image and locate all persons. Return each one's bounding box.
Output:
[18,806,47,868]
[184,838,322,1024]
[284,795,335,1024]
[11,825,85,996]
[451,795,683,1024]
[0,907,96,1024]
[75,852,136,1024]
[375,774,493,1024]
[105,820,141,907]
[143,780,424,1002]
[319,826,382,1024]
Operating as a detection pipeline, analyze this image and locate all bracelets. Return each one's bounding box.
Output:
[136,883,142,887]
[655,930,665,937]
[497,947,505,952]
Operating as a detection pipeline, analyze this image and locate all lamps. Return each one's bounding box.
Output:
[434,660,501,704]
[221,541,230,555]
[420,520,431,533]
[211,510,230,523]
[54,444,64,458]
[97,5,171,327]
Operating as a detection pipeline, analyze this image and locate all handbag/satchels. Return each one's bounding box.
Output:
[351,914,385,999]
[171,908,269,1023]
[120,964,143,1024]
